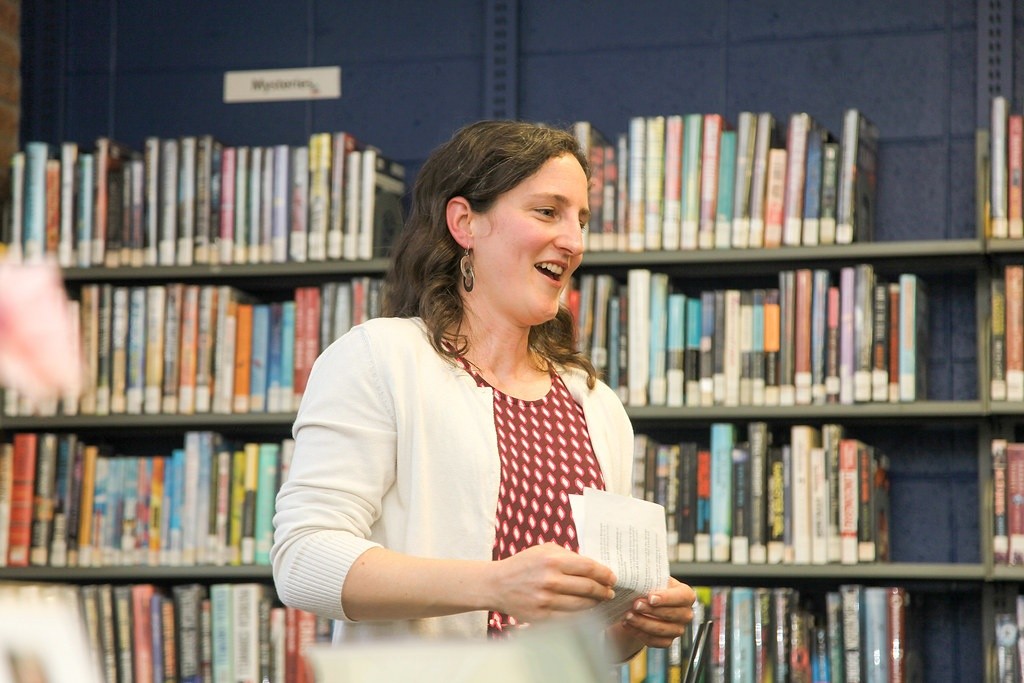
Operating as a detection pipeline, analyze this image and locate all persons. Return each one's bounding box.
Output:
[267,119,697,683]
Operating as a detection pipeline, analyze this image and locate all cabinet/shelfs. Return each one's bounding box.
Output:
[2,237,1024,683]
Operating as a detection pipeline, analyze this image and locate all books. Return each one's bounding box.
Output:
[0,92,1024,683]
